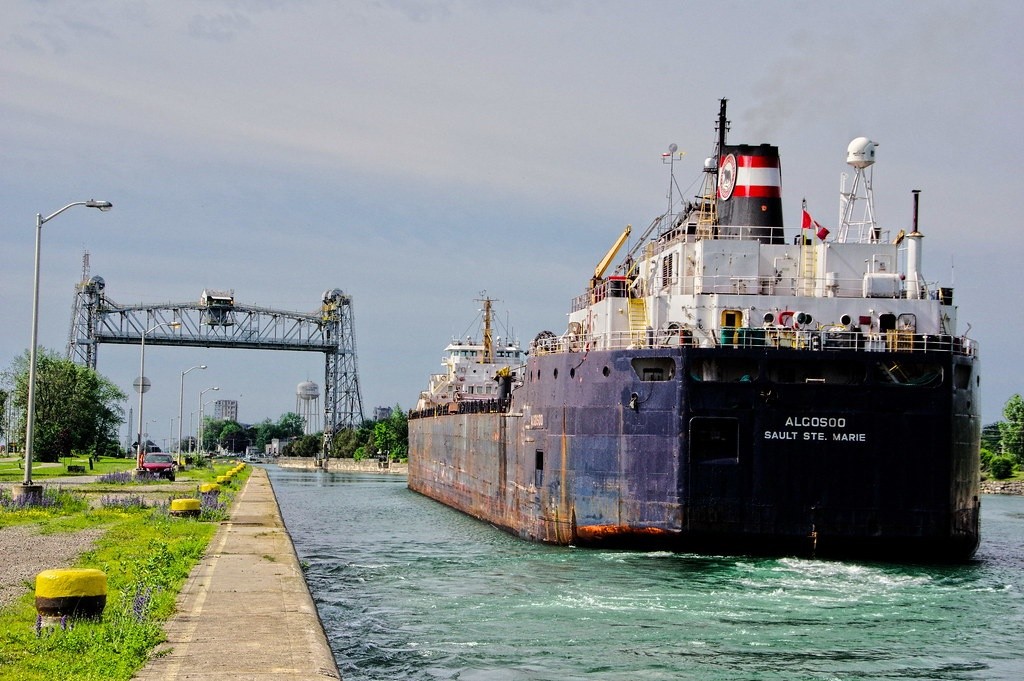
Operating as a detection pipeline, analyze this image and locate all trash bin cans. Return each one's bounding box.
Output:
[680,331,692,348]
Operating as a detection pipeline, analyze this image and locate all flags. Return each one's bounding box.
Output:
[802,209,831,241]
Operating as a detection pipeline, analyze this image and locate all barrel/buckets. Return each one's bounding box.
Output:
[680,330,693,348]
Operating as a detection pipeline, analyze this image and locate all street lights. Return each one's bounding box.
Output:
[178,365,208,465]
[189,409,204,458]
[198,387,219,461]
[22,200,112,488]
[145,420,156,458]
[202,399,218,461]
[136,322,183,470]
[169,415,180,452]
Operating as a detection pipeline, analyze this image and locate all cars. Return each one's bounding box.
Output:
[142,451,178,481]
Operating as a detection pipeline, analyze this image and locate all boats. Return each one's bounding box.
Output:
[243,455,262,464]
[407,98,982,568]
